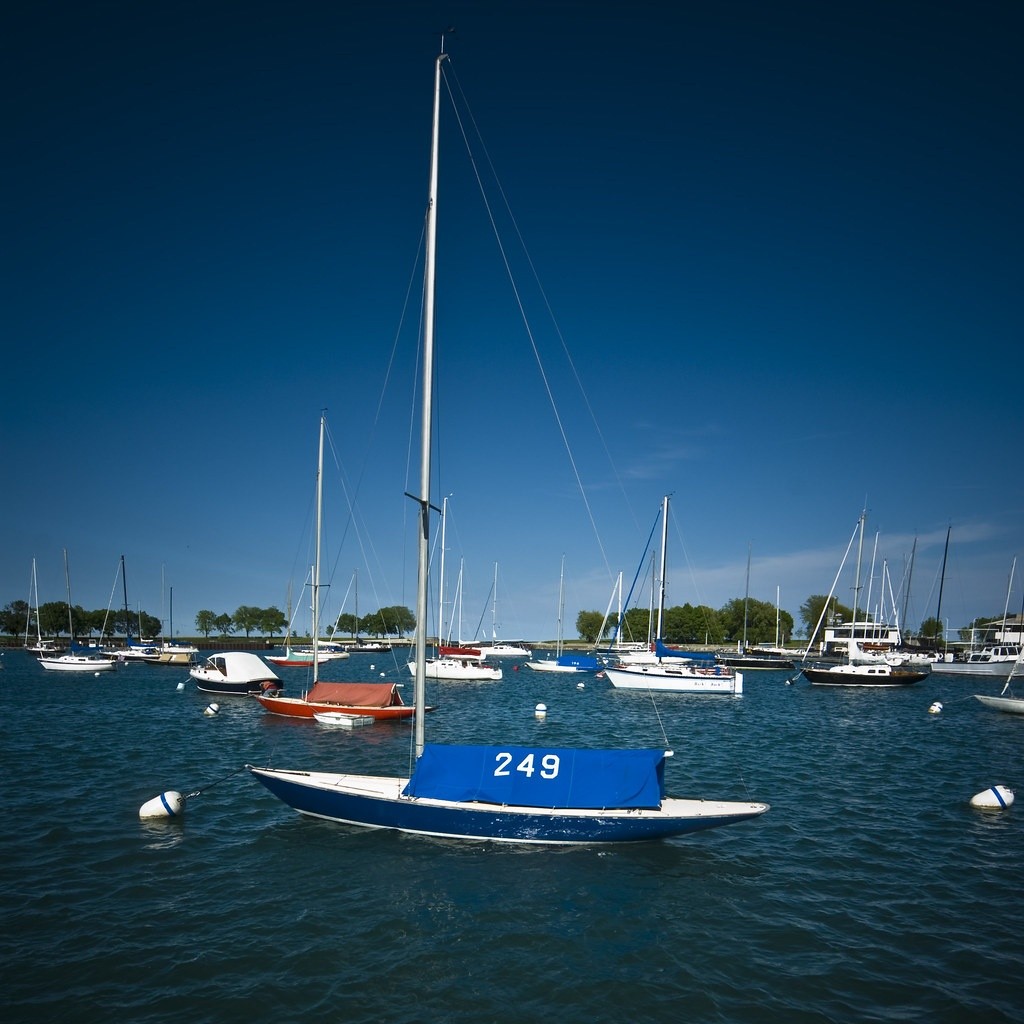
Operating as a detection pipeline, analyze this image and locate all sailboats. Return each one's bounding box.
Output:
[242,38,774,846]
[246,406,440,719]
[972,643,1024,713]
[20,487,1024,698]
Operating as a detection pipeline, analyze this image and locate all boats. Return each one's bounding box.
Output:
[188,651,288,695]
[313,711,376,726]
